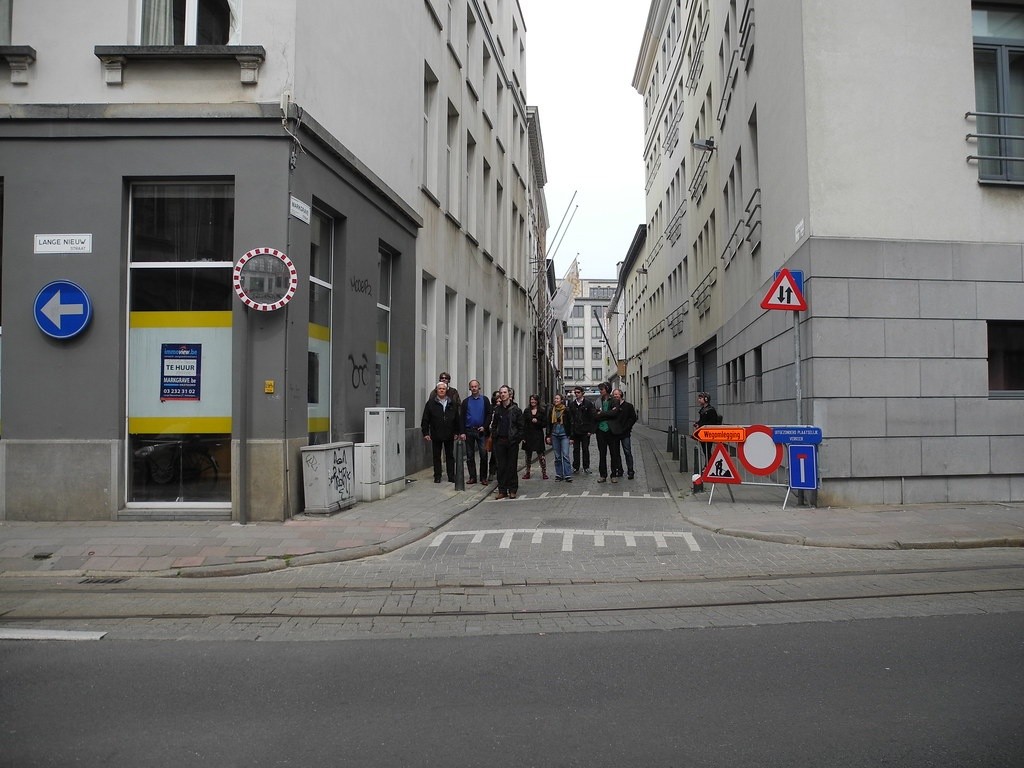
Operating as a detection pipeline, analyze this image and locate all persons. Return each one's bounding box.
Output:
[429,372,638,499]
[420,383,459,482]
[693,392,718,473]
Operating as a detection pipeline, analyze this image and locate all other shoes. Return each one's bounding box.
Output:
[482,479,488,485]
[434,476,440,483]
[597,476,606,483]
[566,477,573,481]
[510,488,517,498]
[466,478,477,484]
[584,468,592,474]
[573,468,579,473]
[555,477,564,482]
[628,472,634,479]
[611,477,618,483]
[522,472,530,479]
[495,489,508,499]
[543,472,548,479]
[488,474,493,481]
[448,475,456,483]
[616,469,624,477]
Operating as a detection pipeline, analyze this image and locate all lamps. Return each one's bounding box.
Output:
[636,268,647,274]
[692,138,717,151]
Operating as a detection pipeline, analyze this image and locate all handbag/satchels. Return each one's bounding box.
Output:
[484,432,493,452]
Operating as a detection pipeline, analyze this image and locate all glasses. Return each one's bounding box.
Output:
[440,377,448,379]
[575,392,580,394]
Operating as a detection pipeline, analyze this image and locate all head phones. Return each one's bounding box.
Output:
[700,390,709,403]
[435,382,450,392]
[619,389,623,397]
[603,382,610,392]
[438,374,451,382]
[468,380,481,390]
